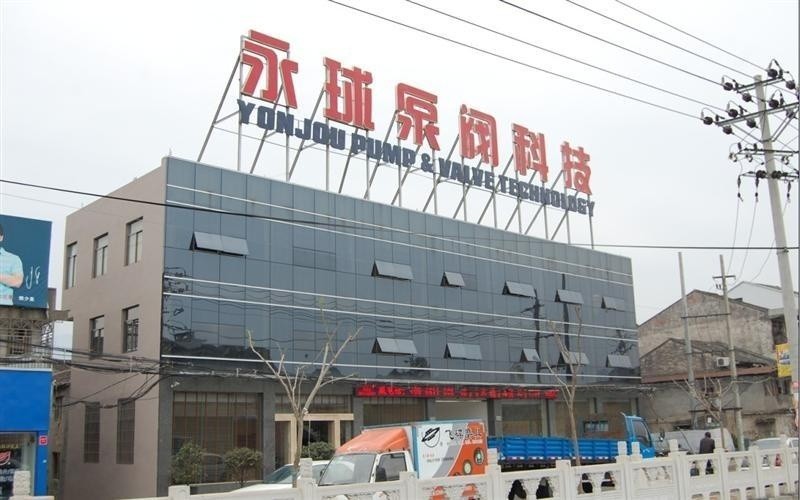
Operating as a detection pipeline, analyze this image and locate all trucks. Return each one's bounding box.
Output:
[315,417,489,499]
[488,409,655,499]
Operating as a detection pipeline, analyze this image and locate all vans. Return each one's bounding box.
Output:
[648,427,736,477]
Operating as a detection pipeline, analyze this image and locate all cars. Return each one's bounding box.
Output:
[741,437,800,467]
[228,461,354,493]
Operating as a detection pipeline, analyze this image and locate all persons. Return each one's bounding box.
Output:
[0,225,24,305]
[699,433,715,466]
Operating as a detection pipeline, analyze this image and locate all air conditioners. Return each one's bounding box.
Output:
[717,357,730,368]
